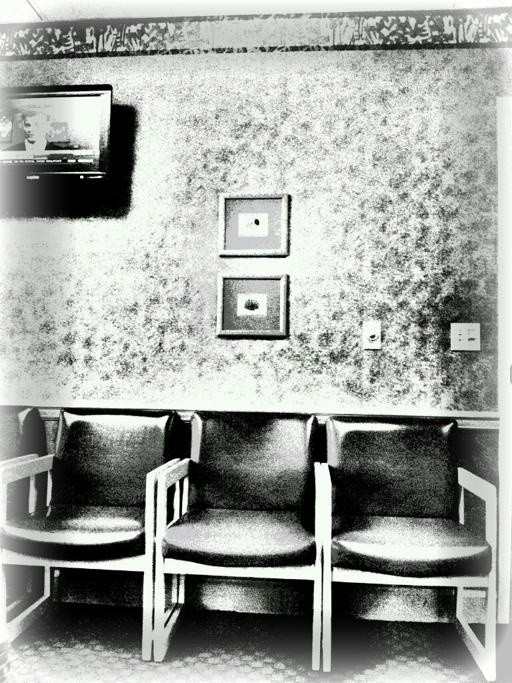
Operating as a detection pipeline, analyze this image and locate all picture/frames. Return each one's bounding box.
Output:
[214,191,291,339]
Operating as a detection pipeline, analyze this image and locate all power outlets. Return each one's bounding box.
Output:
[449,322,481,352]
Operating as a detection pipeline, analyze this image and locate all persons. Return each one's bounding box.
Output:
[2,109,65,151]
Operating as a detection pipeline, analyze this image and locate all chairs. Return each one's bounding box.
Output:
[321,412,499,675]
[1,405,177,663]
[152,409,321,674]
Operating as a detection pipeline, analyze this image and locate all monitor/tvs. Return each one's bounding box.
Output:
[0,83,114,177]
